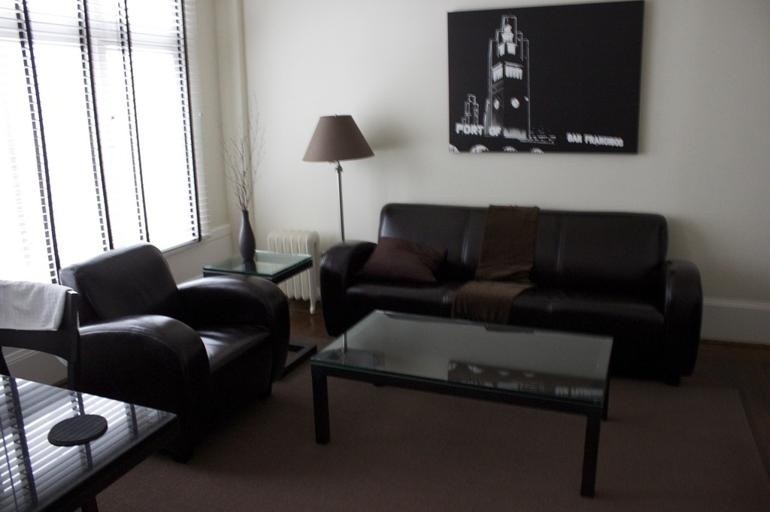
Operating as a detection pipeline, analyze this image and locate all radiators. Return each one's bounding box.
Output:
[266,228,323,315]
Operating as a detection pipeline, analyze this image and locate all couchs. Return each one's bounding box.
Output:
[74,244,288,463]
[321,204,702,386]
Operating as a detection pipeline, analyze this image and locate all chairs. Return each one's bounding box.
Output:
[1,282,102,512]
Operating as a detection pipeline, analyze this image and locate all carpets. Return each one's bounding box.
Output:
[95,368,768,512]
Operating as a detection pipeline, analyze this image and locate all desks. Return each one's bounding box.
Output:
[0,374,177,512]
[202,251,318,383]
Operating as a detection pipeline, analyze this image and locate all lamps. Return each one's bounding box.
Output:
[302,115,375,243]
